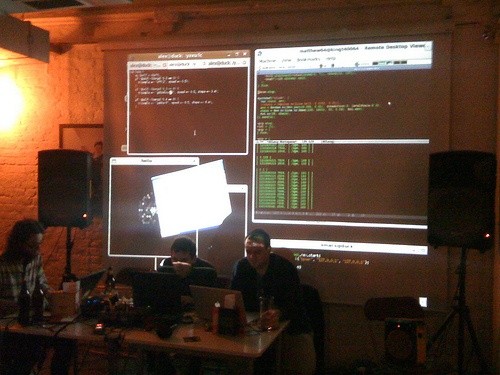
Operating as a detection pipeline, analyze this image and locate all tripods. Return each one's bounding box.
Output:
[426,247,488,375]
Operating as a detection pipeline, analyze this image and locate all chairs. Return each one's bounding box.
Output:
[364,296,430,362]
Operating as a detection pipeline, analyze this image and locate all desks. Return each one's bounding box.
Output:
[0,311,292,375]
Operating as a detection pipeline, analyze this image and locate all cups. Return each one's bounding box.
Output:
[259,295,274,318]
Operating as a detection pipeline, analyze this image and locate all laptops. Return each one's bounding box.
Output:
[43,269,105,318]
[188,285,263,325]
[131,271,182,310]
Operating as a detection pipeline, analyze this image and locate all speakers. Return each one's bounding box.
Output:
[37,149,96,228]
[427,151,495,252]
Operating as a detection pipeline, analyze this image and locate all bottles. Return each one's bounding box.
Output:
[18,279,32,324]
[104,264,118,294]
[31,277,46,321]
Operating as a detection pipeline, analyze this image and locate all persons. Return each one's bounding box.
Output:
[231,230,314,375]
[0,219,75,375]
[146,237,218,375]
